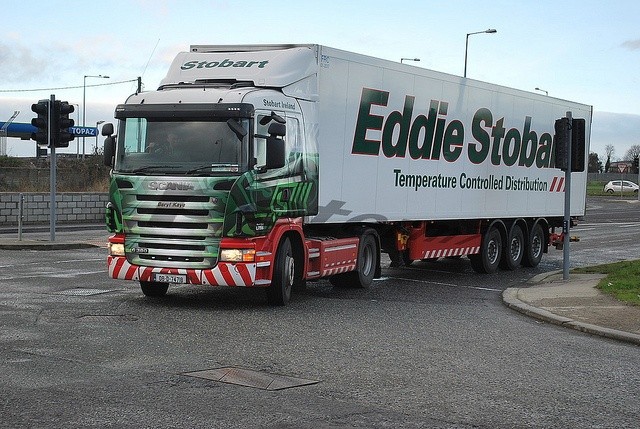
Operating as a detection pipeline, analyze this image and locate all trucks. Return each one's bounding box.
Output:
[102,43,593,308]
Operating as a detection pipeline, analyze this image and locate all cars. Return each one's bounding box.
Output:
[603,179,640,194]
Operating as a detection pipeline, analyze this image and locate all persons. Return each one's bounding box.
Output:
[148,128,175,156]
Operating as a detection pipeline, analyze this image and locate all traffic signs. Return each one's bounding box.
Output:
[618,164,627,173]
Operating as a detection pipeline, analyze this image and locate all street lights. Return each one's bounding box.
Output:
[72,103,79,161]
[535,87,549,97]
[96,120,106,155]
[83,75,110,157]
[464,29,497,79]
[400,58,420,67]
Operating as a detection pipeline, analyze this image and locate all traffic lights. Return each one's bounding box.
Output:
[572,119,585,172]
[32,99,50,147]
[54,100,75,147]
[555,118,568,171]
